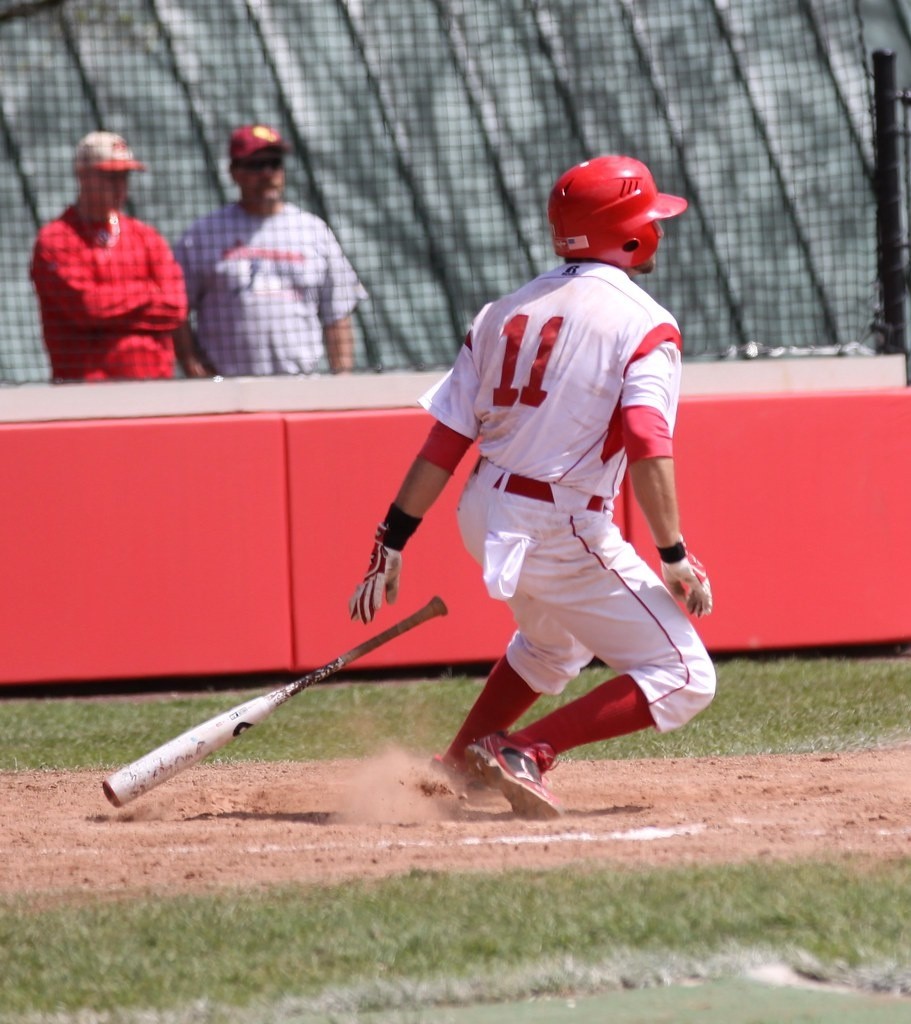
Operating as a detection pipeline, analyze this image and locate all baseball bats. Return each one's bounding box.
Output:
[99,593,450,811]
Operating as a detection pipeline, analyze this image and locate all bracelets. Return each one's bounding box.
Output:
[383,503,422,535]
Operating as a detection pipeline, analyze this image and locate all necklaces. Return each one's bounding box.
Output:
[81,210,120,247]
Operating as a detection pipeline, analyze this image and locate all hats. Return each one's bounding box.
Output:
[230,125,292,162]
[71,130,147,177]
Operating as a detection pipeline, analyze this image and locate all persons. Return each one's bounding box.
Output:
[171,126,365,376]
[351,157,716,821]
[32,129,186,382]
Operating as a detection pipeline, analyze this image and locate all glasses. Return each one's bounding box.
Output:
[233,158,282,170]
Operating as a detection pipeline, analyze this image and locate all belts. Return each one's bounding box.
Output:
[474,458,604,514]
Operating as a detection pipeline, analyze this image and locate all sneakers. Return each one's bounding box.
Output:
[422,753,498,796]
[465,729,564,821]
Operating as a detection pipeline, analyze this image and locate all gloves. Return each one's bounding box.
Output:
[348,502,423,626]
[655,532,713,619]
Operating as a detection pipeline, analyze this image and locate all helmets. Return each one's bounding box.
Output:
[547,155,689,269]
